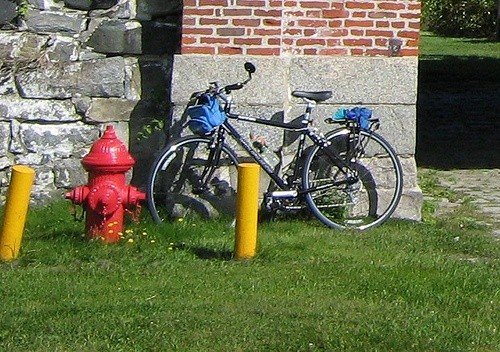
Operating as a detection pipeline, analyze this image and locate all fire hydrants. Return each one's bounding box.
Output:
[64,124,146,241]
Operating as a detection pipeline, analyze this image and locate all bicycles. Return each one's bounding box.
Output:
[147,62,403,237]
